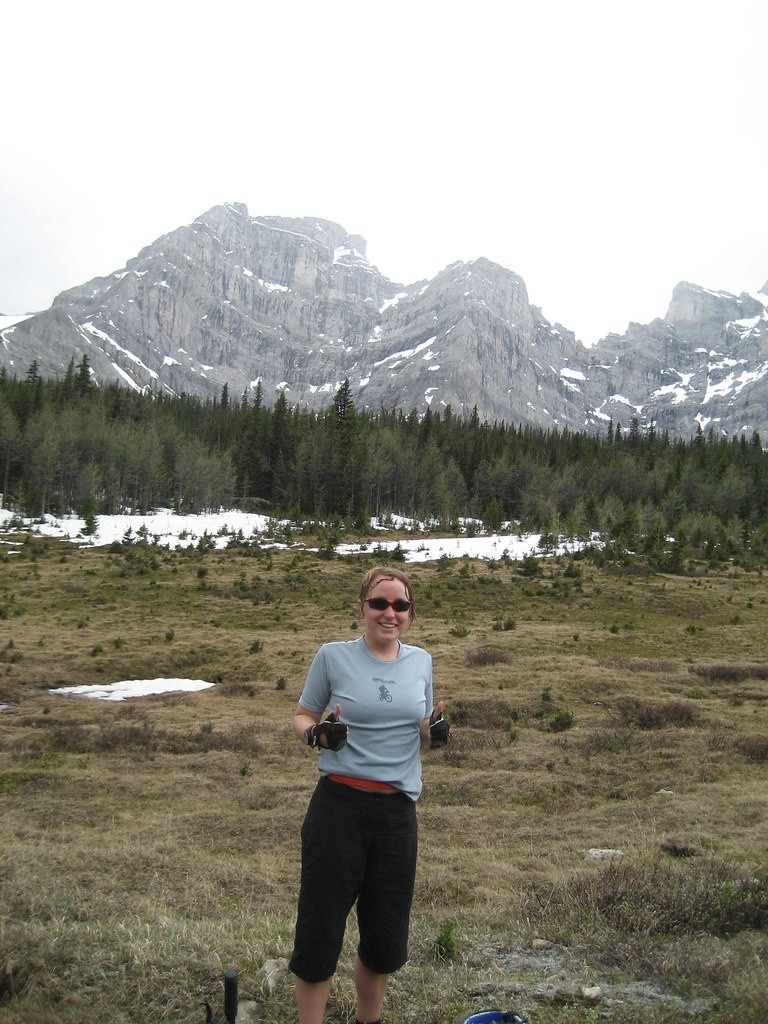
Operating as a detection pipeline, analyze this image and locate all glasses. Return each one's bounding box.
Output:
[365,598,411,612]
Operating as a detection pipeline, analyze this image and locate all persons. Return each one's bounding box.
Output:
[287,565,451,1024]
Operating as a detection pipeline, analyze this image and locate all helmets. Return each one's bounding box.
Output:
[462,1009,526,1024]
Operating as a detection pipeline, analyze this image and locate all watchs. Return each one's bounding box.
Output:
[302,723,317,748]
